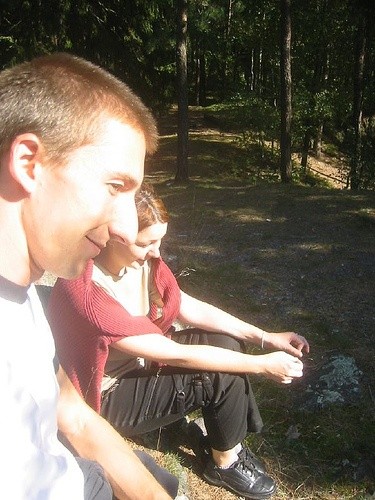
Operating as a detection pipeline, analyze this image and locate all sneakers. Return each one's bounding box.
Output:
[201,456,276,499]
[202,434,267,475]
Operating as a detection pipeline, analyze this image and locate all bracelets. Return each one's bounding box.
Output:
[261,331,267,350]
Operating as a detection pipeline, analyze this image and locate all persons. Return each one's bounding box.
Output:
[44,184,311,500]
[0,48,185,500]
